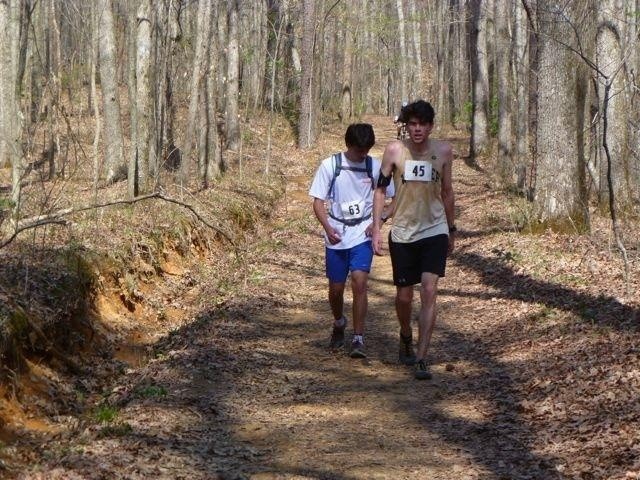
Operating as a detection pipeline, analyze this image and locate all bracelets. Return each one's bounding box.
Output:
[381,213,389,223]
[449,226,457,233]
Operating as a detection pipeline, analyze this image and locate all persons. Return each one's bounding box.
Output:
[310,124,399,360]
[370,98,456,378]
[394,103,408,141]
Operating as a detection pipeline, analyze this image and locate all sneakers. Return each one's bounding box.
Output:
[398,326,434,380]
[330,314,368,358]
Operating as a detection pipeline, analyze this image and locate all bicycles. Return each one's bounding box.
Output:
[395,120,407,141]
